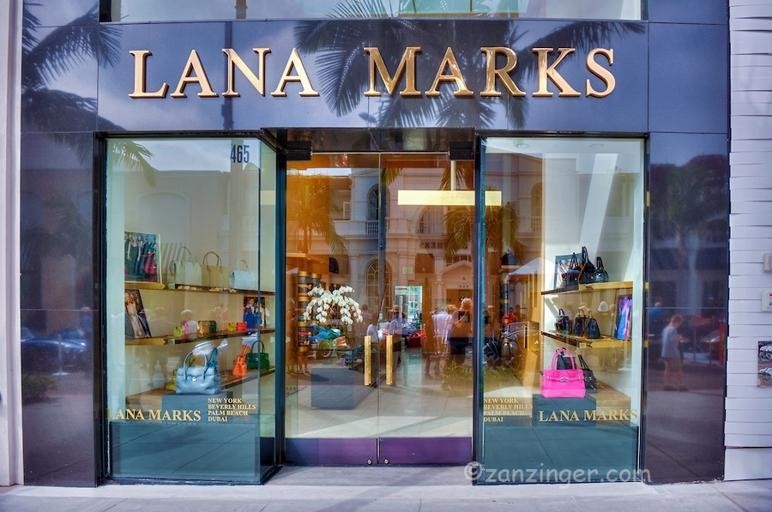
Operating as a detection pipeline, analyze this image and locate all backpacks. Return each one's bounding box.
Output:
[164,346,224,396]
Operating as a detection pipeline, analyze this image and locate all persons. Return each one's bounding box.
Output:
[124,233,157,281]
[286,296,312,376]
[422,297,520,380]
[137,304,201,390]
[658,312,690,392]
[78,305,94,381]
[647,301,665,369]
[124,290,152,340]
[612,296,632,341]
[356,298,412,389]
[244,298,266,328]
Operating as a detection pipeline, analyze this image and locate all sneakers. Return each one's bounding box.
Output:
[662,381,689,393]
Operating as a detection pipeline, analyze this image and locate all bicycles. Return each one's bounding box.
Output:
[445,344,504,400]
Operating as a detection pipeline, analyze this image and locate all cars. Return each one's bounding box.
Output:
[648,308,724,368]
[21,325,90,373]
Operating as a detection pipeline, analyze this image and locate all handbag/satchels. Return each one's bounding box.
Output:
[560,251,581,286]
[537,347,599,399]
[231,343,252,377]
[554,306,575,335]
[201,250,231,289]
[246,339,270,370]
[571,308,602,339]
[577,246,596,284]
[172,317,249,338]
[593,255,609,282]
[166,246,203,287]
[230,258,260,291]
[500,248,518,266]
